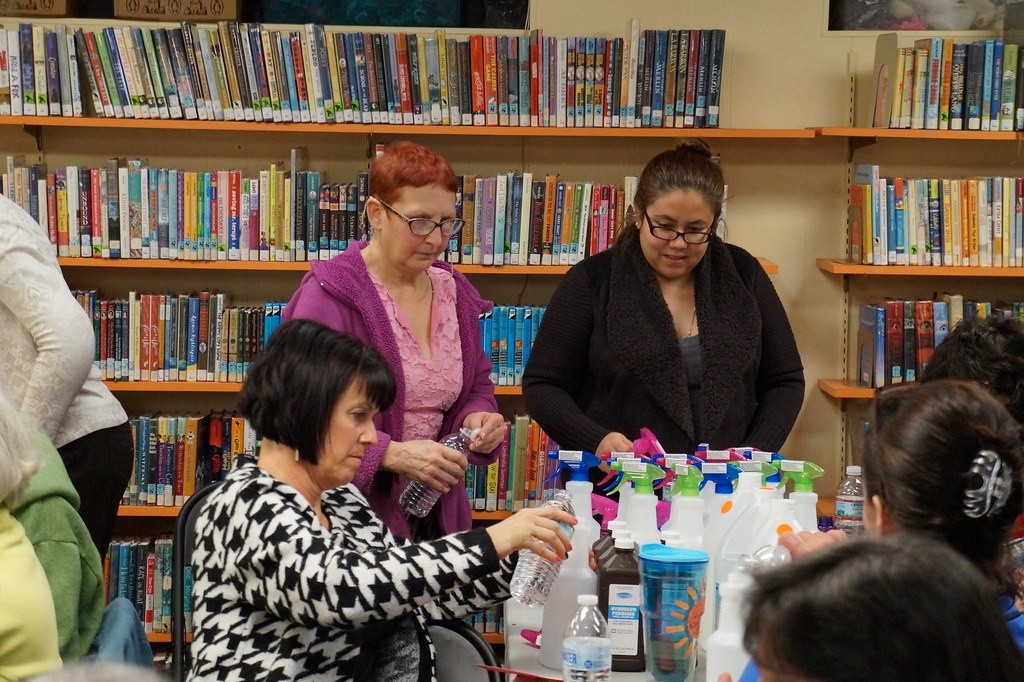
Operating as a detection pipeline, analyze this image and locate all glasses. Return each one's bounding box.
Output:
[643,205,718,245]
[376,198,466,236]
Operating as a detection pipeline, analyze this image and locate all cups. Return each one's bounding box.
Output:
[636,543,709,682]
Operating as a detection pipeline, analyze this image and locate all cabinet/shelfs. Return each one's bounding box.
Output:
[0,116,815,643]
[807,125,1023,520]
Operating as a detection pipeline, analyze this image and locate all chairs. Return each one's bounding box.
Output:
[171,480,507,682]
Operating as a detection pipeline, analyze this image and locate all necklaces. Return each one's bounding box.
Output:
[670,305,698,336]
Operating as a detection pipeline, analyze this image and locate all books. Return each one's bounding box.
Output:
[0,20,283,123]
[147,162,270,262]
[855,164,1024,268]
[72,290,288,383]
[859,292,1024,389]
[184,565,193,631]
[450,28,631,128]
[0,153,149,259]
[874,37,1024,131]
[464,411,565,513]
[103,532,173,633]
[282,23,450,126]
[437,171,728,265]
[479,304,549,385]
[270,144,385,262]
[634,29,727,126]
[119,408,261,507]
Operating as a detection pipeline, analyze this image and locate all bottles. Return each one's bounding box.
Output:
[399,427,480,518]
[509,489,565,607]
[562,594,612,682]
[833,466,866,538]
[733,544,798,577]
[590,520,647,674]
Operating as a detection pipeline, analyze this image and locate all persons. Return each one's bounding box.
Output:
[0,192,134,558]
[282,138,806,547]
[738,377,1024,682]
[184,317,578,682]
[919,308,1024,571]
[0,396,103,665]
[737,531,1024,682]
[0,503,64,682]
